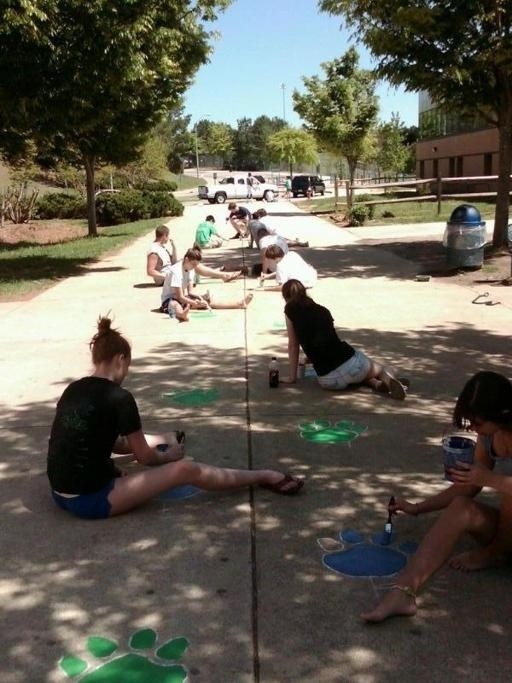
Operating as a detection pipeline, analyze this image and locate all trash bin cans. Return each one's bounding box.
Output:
[447,204,486,268]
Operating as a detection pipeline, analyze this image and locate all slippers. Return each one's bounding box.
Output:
[156,430,185,452]
[271,472,304,496]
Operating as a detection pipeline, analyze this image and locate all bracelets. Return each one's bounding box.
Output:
[414,502,420,518]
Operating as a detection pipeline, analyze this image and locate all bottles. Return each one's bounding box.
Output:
[297,364,305,379]
[248,267,252,276]
[269,357,280,388]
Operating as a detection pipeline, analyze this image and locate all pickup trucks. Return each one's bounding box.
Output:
[196,175,280,204]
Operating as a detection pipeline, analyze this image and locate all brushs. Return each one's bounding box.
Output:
[382,496,396,544]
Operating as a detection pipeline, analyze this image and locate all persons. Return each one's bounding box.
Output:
[360,370,512,627]
[281,175,293,201]
[246,172,259,199]
[46,306,303,521]
[304,177,314,199]
[145,201,318,322]
[280,277,411,404]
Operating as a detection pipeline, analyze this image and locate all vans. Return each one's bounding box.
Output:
[291,175,326,198]
